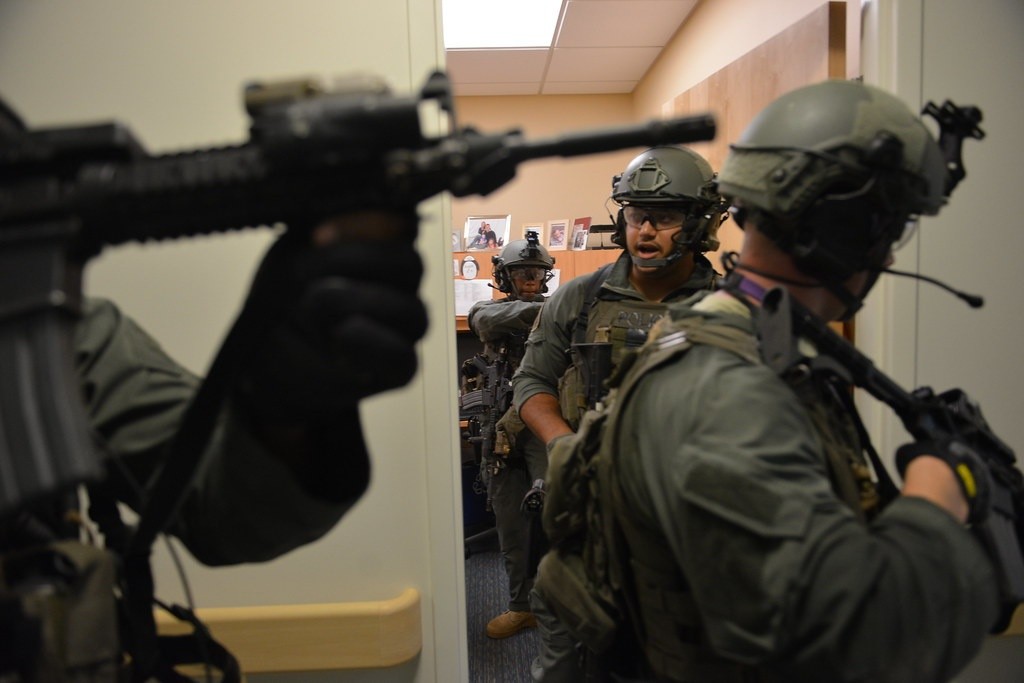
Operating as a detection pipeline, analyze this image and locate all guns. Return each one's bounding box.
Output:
[461,337,516,513]
[566,341,616,414]
[1,59,720,525]
[757,280,1024,612]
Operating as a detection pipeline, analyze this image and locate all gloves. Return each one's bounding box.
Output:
[893,384,993,528]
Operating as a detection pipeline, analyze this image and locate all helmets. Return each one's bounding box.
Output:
[716,79,947,239]
[491,230,556,270]
[611,144,722,202]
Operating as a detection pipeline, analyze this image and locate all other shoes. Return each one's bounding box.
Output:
[484,609,537,638]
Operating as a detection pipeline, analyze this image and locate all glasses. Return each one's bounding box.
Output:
[511,267,545,280]
[870,208,918,251]
[621,203,696,232]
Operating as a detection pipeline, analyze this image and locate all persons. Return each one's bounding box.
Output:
[0,94,430,683]
[466,238,557,636]
[512,145,723,453]
[467,221,503,250]
[551,228,564,246]
[615,80,1001,682]
[576,232,586,248]
